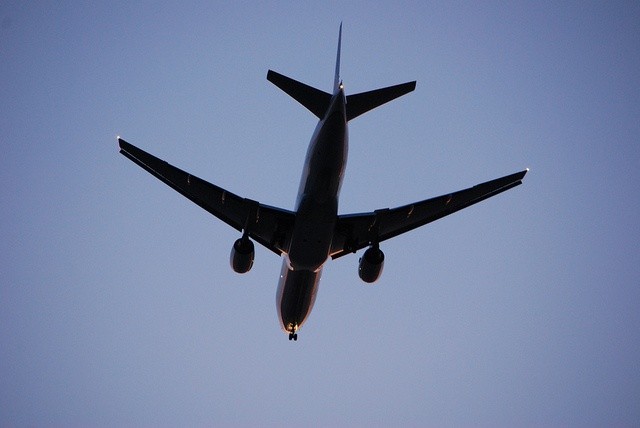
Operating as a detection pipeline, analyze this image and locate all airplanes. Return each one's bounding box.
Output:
[117,20,530,342]
